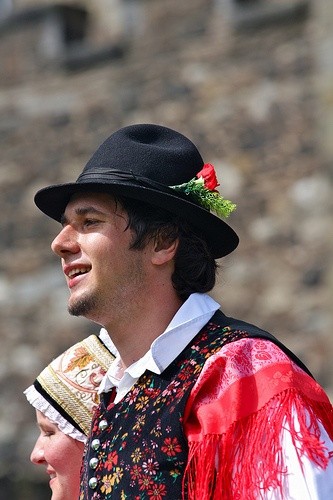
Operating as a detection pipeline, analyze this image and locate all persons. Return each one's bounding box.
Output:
[23,328,120,500]
[34,123,333,500]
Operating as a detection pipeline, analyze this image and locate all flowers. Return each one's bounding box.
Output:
[169,163,237,218]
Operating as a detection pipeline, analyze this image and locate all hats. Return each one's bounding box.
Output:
[34,123,240,260]
[23,328,119,444]
